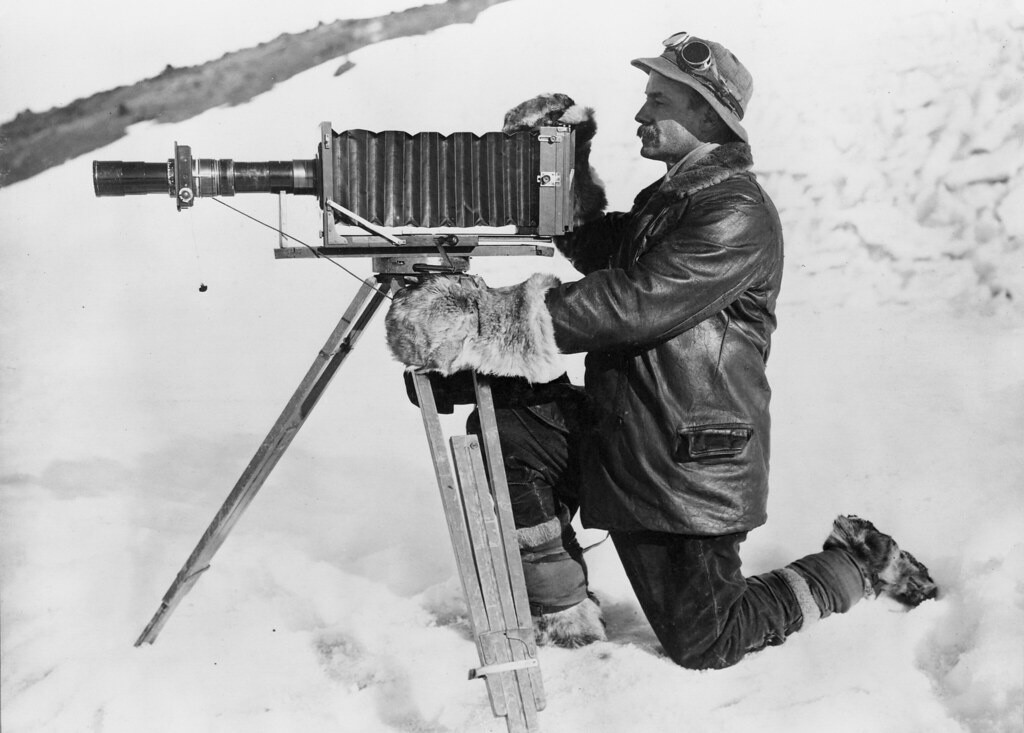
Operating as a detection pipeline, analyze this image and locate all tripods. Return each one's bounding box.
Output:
[133,254,547,733]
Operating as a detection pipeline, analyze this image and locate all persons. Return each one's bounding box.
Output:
[383,31,940,670]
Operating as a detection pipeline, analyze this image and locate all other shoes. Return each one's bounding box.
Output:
[824,515,941,607]
[533,606,608,649]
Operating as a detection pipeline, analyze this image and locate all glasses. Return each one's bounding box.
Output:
[661,31,720,80]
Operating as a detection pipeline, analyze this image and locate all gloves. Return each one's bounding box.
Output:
[504,92,607,218]
[385,273,564,383]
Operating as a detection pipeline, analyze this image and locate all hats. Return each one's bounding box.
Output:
[630,37,754,143]
